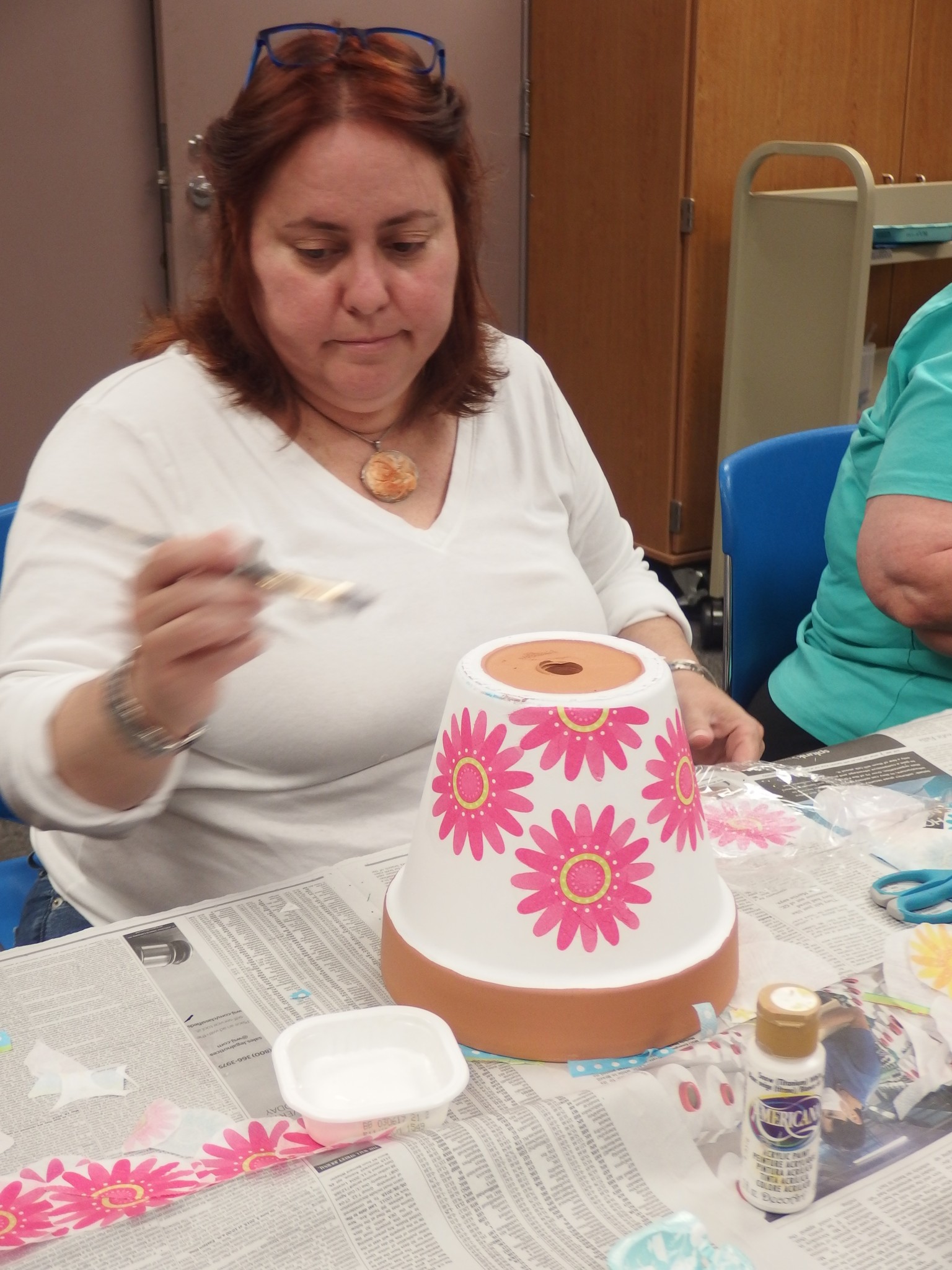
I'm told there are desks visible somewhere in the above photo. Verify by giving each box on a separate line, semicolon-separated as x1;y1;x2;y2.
0;708;951;1270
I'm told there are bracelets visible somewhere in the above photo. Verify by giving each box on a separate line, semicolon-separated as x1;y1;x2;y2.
106;645;209;756
668;659;719;687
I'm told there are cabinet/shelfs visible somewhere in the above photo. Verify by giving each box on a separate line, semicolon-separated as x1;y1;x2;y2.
523;0;952;567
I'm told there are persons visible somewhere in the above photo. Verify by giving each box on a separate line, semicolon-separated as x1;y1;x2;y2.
752;289;952;761
814;991;883;1151
0;18;768;978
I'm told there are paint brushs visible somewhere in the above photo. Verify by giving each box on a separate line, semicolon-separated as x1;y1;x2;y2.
23;499;376;616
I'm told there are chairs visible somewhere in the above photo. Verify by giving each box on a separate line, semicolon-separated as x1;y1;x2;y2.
719;424;858;759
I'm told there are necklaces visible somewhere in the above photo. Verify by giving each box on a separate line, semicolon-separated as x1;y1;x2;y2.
298;389;423;507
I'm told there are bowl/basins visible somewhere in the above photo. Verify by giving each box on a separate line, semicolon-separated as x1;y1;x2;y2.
271;1005;472;1149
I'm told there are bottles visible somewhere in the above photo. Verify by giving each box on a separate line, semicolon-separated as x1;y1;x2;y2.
739;981;827;1215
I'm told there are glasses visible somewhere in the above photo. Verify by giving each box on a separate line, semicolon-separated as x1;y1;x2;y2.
241;24;448;94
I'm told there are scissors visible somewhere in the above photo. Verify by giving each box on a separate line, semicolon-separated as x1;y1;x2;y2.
869;861;951;924
